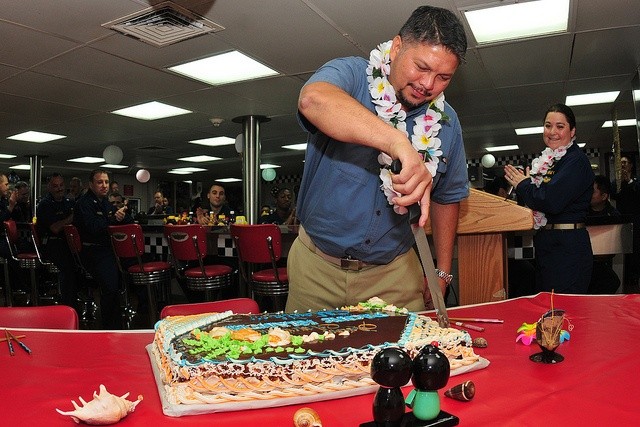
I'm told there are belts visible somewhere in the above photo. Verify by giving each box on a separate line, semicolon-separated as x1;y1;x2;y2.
543;223;586;231
298;224;373;271
49;236;63;239
81;242;101;247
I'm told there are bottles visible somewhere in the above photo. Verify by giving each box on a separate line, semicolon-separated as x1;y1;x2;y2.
269;209;278;225
229;210;236;223
123;199;129;224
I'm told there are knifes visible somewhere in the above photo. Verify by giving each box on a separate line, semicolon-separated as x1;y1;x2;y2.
390;159;452;330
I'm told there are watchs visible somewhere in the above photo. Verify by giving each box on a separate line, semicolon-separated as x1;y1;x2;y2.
433;268;455;285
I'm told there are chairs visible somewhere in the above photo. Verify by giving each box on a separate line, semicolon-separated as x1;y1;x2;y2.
30;224;61;274
162;226;233;302
2;220;39;306
228;223;290;311
158;297;260;322
108;224;170;327
0;304;79;329
64;223;95;281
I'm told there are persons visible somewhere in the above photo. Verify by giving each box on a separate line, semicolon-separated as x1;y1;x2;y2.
79;170;126;307
193;183;237;225
0;176;18;225
591;176;621;294
36;174;75;262
149;190;167;218
108;192;132;216
620;156;633;184
283;4;471;314
269;185;300;232
504;106;597;296
259;206;272;215
13;180;33;241
109;180;120;194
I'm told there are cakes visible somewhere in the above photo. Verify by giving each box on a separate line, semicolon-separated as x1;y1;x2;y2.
146;295;490;417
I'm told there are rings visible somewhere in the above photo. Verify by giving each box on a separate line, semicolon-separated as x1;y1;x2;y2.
509;176;514;180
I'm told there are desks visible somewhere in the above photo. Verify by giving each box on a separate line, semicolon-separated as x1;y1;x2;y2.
0;287;640;427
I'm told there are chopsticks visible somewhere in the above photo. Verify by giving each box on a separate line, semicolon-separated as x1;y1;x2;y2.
431;314;507;331
0;327;31;357
